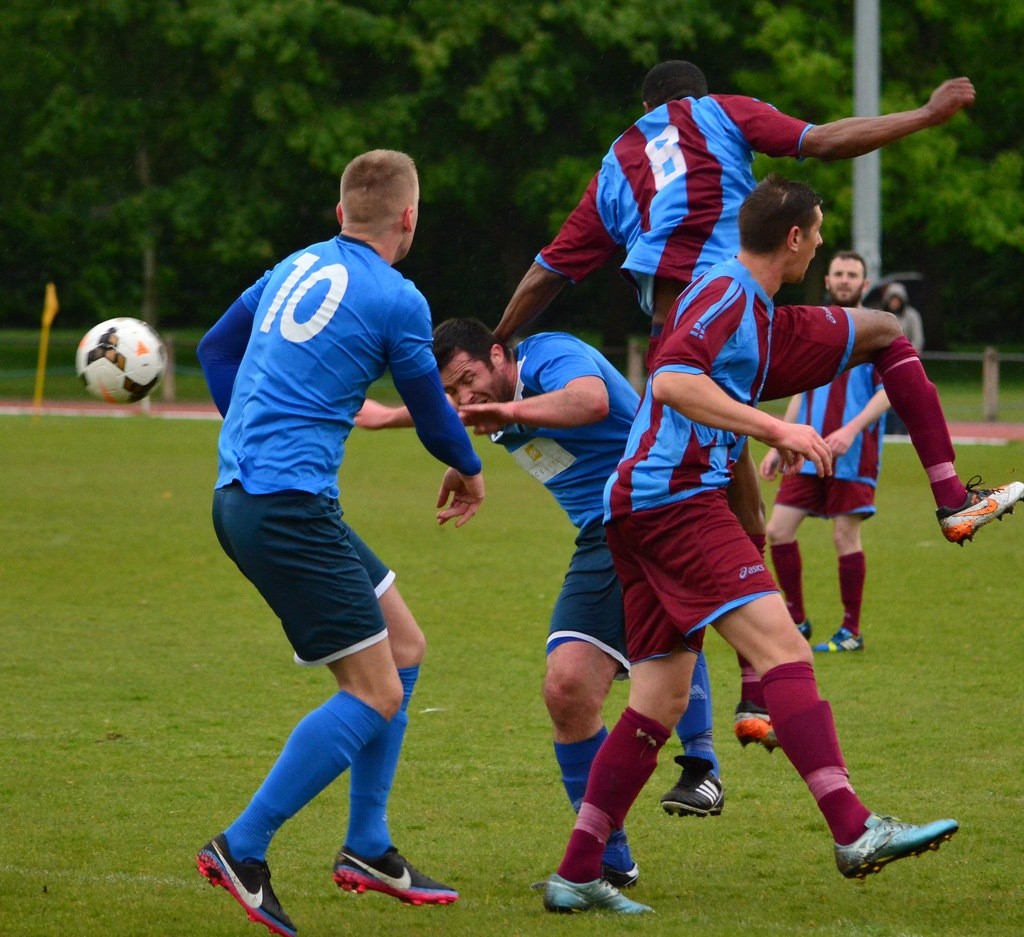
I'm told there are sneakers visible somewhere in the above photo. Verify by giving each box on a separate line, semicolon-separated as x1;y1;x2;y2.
792;621;812;640
333;844;458;906
543;872;657;914
812;626;862;656
937;481;1024;543
734;701;782;753
529;863;640;890
198;832;298;937
662;755;725;817
833;811;959;881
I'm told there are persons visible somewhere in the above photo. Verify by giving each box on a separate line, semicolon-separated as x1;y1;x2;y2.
187;149;484;937
879;282;924;435
489;56;1022;759
548;169;968;917
758;248;897;656
351;314;729;898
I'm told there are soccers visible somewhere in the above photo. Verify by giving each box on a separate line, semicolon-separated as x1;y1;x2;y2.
74;316;167;405
